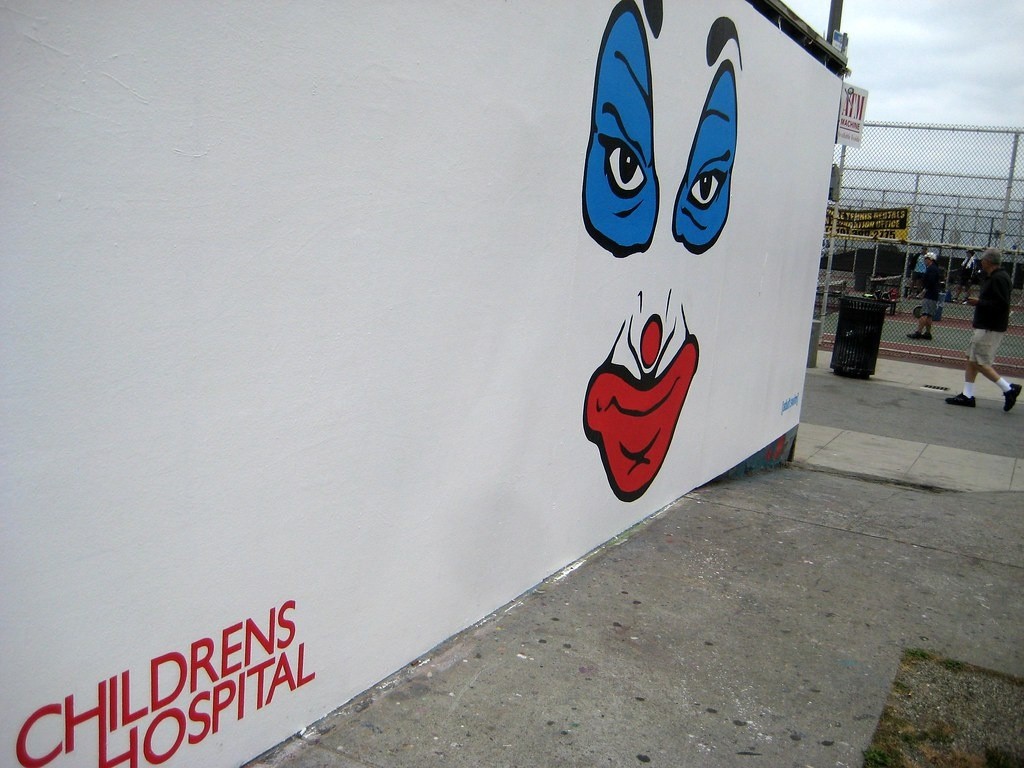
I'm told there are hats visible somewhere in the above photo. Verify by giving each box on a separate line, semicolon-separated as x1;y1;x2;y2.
921;252;937;261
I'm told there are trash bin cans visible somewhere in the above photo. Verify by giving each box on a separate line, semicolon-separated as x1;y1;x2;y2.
829;294;890;381
932;293;946;321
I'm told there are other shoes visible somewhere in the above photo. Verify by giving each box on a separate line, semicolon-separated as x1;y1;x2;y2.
961;300;967;305
952;299;958;303
921;333;932;340
907;332;921;339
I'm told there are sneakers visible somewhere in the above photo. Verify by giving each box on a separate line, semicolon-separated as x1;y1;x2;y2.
1004;383;1022;411
945;393;975;407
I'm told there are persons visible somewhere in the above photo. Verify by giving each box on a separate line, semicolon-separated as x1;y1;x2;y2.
905;246;940;341
944;248;1022;413
